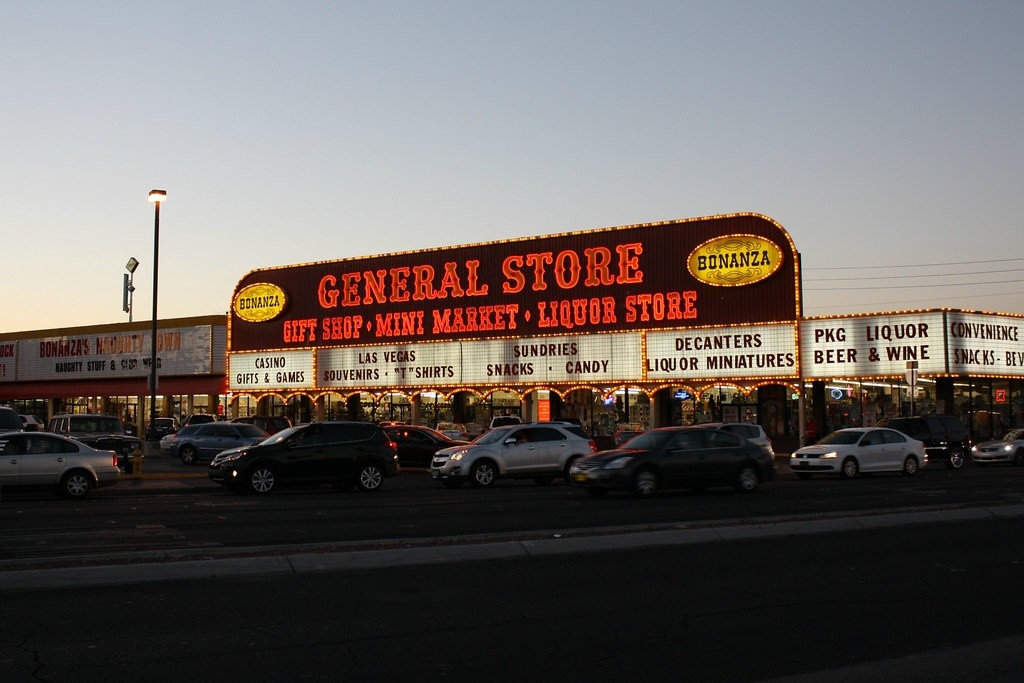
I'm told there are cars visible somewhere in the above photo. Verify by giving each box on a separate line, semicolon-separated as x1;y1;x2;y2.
379;416;596;489
0;406;142;499
146;413;294;466
790;427;928;480
971;429;1024;464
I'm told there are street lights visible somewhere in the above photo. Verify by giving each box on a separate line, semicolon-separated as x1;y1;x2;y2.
144;189;168;457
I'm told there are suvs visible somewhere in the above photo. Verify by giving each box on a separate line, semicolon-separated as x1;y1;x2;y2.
699;423;776;462
872;415;972;469
568;420;776;500
211;421;400;498
